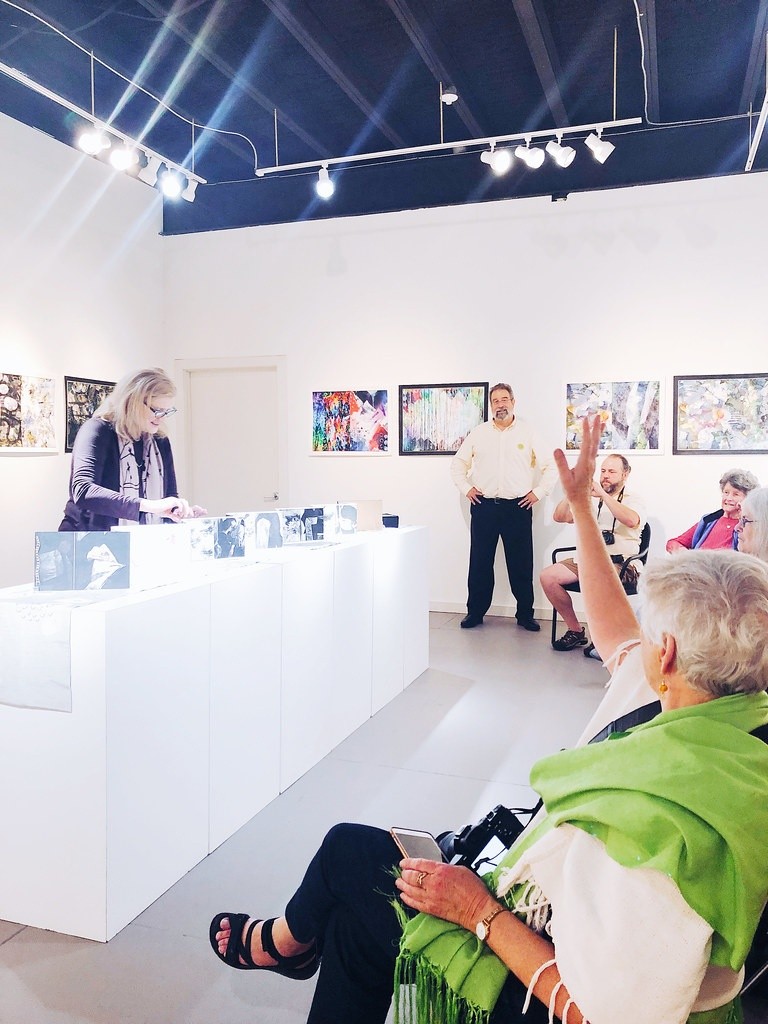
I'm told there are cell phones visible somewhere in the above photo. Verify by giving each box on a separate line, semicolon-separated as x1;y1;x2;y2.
389;827;451;864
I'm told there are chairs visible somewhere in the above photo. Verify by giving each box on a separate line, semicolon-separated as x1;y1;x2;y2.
551;522;651;657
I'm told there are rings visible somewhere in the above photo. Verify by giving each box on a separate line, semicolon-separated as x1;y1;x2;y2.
416;872;427;886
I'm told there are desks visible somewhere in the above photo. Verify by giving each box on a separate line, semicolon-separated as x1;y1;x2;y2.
0;520;432;944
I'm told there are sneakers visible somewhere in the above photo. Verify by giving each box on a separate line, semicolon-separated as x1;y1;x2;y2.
553;626;588;651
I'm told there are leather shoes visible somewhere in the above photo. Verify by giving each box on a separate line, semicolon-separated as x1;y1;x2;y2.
461;614;483;628
517;617;540;631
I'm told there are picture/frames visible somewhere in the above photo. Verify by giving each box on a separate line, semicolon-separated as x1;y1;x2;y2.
0;371;62;453
399;382;489;456
64;376;118;453
673;373;768;454
307;387;394;457
562;376;665;456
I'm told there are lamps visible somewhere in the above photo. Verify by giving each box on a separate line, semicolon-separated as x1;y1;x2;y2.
442;83;459;106
515;137;545;168
78;124;111;154
111;141;139;169
545;133;577;168
584;127;617;164
315;165;334;198
480;142;511;172
162;168;181;198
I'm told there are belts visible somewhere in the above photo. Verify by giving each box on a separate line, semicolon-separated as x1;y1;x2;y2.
478;497;525;504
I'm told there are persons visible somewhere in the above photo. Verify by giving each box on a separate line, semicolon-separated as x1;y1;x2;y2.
59;368;207;531
461;383;560;631
212;416;768;1024
218;520;245;557
538;453;645;652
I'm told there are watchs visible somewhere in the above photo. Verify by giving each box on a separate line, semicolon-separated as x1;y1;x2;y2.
475;907;508;942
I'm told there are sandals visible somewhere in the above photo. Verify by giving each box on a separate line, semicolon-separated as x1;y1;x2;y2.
209;912;320;980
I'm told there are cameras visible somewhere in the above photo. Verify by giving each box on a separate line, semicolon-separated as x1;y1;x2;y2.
602;530;614;544
434;805;526;878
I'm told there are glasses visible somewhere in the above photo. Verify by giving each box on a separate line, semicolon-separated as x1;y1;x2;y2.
491;398;513;404
143;401;177;419
739;518;756;527
